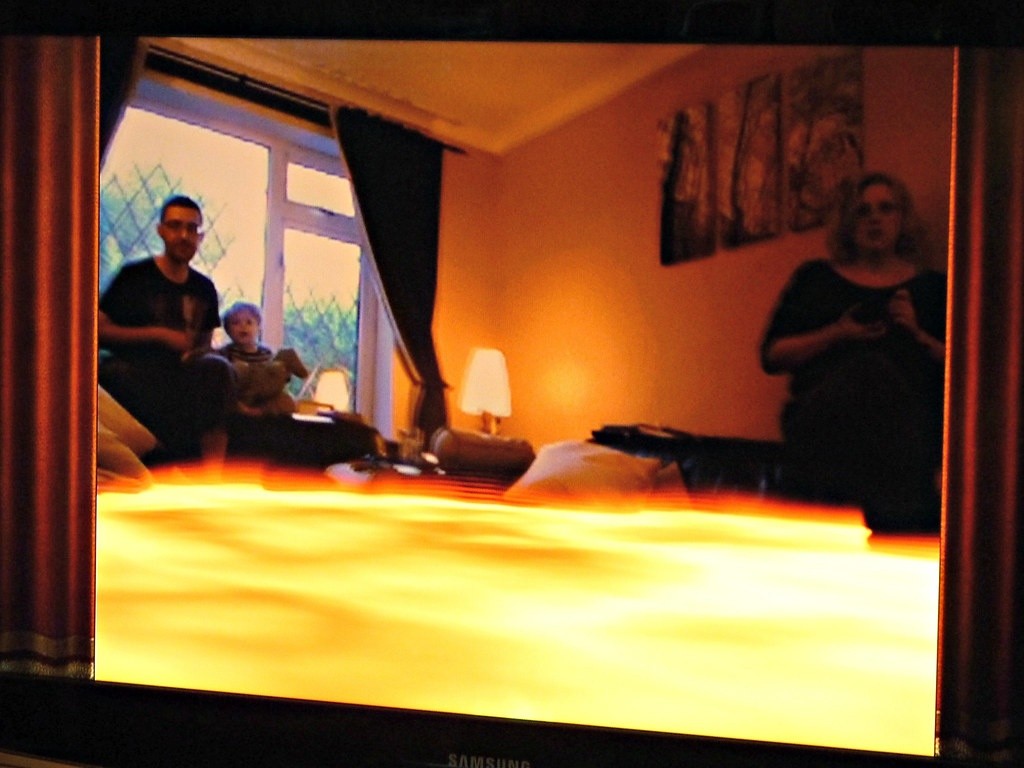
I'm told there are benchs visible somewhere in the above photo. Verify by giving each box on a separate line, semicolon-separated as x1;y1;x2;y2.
595;431;795;497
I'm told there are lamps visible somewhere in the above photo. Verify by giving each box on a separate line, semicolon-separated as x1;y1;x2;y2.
313;370;351;414
462;347;510;436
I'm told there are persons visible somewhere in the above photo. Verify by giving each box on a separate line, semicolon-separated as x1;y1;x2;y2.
218;301;294;445
98;194;231;468
759;173;945;503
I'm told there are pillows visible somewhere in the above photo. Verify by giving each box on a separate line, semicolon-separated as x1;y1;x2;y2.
505;441;662;502
